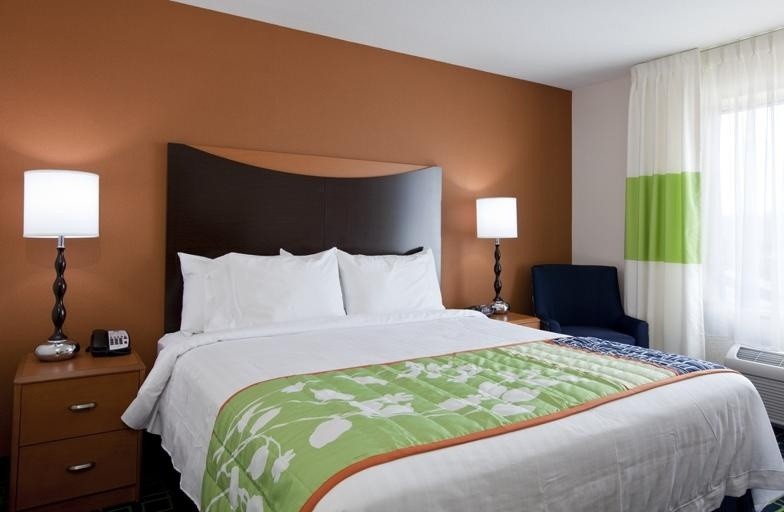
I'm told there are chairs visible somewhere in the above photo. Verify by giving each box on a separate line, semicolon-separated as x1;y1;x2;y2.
530;264;649;349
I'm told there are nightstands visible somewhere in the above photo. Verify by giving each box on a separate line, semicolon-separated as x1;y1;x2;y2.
9;349;148;512
488;311;540;329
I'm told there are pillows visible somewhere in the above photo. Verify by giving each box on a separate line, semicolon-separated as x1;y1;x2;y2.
176;244;447;338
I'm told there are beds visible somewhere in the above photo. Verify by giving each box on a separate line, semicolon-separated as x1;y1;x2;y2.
160;140;763;512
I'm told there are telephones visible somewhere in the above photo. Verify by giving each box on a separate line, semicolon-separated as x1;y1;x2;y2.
90;329;132;357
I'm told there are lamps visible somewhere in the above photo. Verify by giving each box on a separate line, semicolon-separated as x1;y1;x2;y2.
21;168;100;363
475;196;518;314
